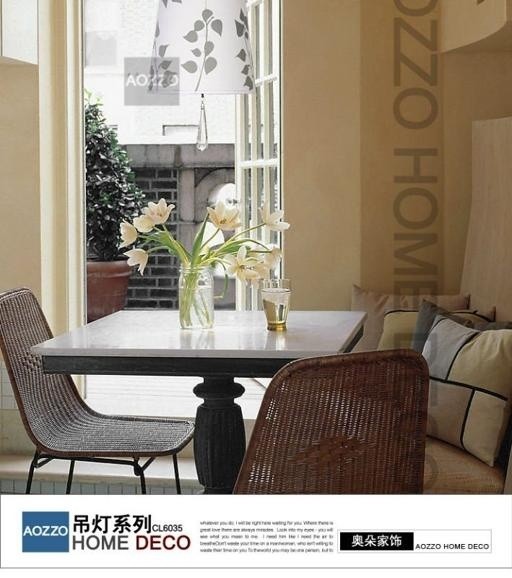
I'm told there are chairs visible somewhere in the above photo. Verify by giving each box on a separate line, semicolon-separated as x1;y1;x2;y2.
0;284;199;494
229;344;430;494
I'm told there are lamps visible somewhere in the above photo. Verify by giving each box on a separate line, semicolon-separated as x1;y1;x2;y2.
141;0;259;152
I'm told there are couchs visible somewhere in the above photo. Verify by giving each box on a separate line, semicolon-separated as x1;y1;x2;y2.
422;432;512;494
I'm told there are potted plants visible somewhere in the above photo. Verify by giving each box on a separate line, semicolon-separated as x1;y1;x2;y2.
81;85;150;321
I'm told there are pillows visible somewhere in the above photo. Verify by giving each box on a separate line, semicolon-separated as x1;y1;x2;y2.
413;313;512;467
345;281;473;354
410;298;512;371
373;304;500;355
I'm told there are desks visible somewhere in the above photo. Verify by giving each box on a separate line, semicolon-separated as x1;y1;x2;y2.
28;306;372;493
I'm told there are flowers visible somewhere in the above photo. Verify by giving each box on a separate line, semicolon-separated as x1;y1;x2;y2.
112;196;292;287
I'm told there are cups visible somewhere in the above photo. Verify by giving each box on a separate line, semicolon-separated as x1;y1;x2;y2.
258;277;292;332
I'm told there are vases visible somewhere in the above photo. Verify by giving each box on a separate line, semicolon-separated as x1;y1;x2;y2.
176;261;216;331
